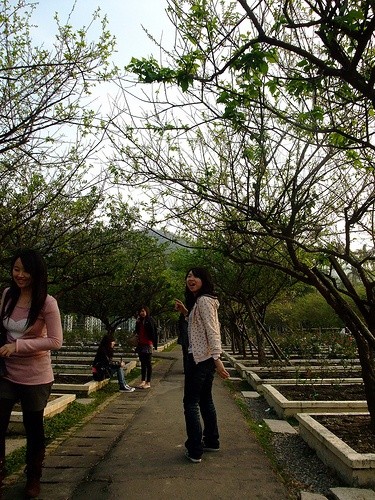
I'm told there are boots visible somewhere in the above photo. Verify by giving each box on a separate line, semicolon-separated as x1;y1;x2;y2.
24;447;45;497
0;456;6;500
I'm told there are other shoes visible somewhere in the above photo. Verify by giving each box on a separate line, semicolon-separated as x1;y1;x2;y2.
201;441;219;452
185;449;201;463
135;384;150;389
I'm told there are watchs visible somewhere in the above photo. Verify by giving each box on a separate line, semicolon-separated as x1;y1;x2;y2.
214;356;220;360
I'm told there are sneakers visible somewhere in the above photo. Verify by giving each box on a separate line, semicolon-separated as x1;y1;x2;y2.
119;386;134;392
125;383;136;390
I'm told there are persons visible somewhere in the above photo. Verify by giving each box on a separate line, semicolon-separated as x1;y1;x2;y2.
175;267;229;463
0;248;63;499
132;306;158;388
178;306;189;373
340;327;352;334
92;334;135;392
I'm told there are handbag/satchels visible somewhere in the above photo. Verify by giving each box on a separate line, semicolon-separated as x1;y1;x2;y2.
93;364;106;381
0;316;7;376
